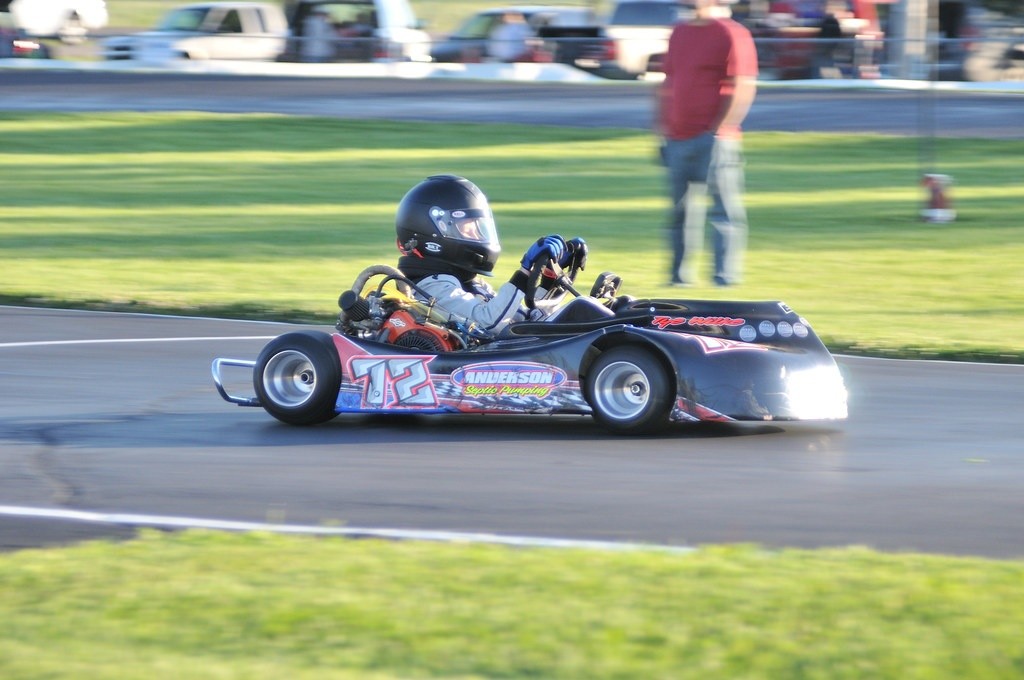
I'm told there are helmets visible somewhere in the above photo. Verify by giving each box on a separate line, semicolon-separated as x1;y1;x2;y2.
397;174;502;276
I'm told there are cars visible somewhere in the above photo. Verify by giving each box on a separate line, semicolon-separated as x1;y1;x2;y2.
274;1;433;63
1;27;51;59
432;4;617;66
103;3;289;61
603;2;704;80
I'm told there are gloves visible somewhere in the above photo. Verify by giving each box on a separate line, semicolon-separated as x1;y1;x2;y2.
520;235;568;272
559;237;588;273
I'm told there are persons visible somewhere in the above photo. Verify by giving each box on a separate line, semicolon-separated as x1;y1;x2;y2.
298;13;348;63
397;173;585;333
657;0;759;292
486;12;538;62
